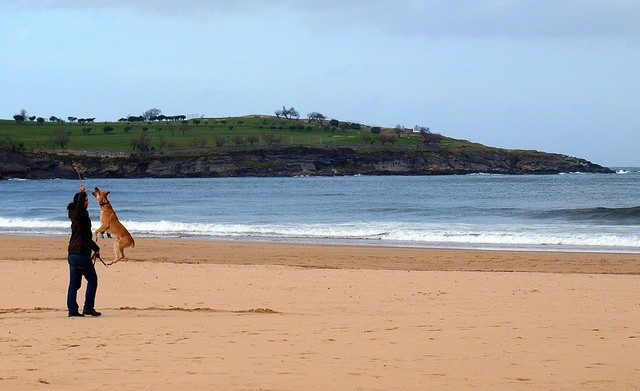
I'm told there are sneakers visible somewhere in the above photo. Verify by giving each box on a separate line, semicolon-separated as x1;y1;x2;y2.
68;311;85;317
83;309;101;316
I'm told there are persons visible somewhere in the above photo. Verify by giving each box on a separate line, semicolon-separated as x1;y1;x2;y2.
67;184;101;317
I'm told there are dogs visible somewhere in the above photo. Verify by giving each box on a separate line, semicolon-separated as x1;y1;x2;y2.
91;186;135;266
